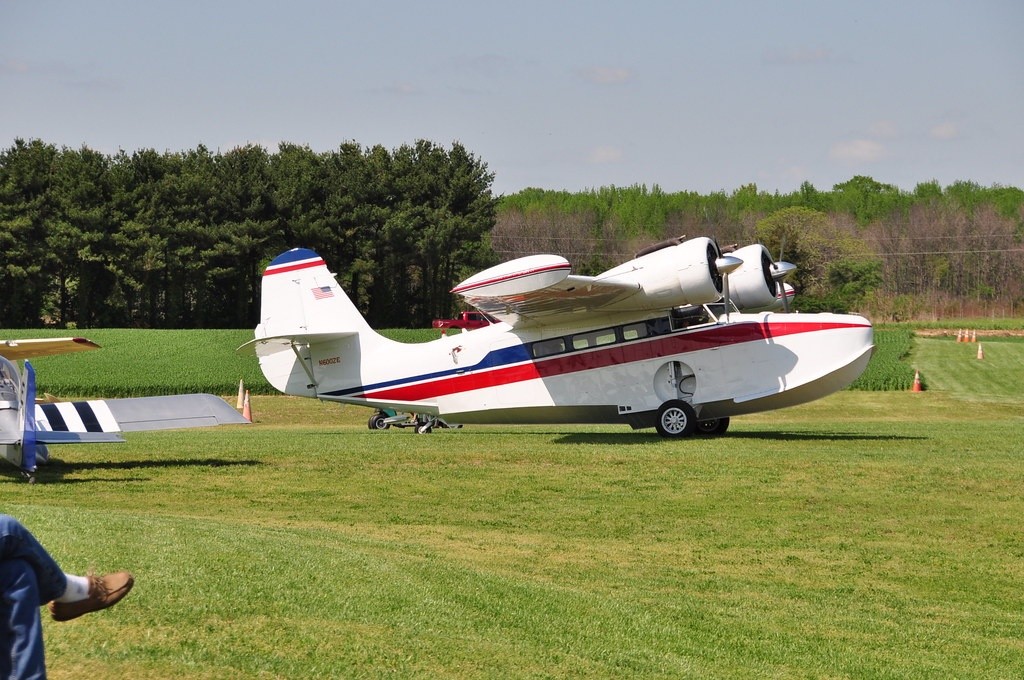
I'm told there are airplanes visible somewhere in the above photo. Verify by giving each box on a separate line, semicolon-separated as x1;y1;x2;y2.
236;234;875;439
0;337;251;484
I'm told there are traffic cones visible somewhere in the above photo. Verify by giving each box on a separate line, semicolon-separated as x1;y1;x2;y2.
971;329;977;342
910;369;924;392
977;343;983;360
957;328;962;342
236;379;246;409
243;390;252;422
964;329;969;342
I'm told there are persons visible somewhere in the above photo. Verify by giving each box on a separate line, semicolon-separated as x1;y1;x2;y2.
0;514;134;680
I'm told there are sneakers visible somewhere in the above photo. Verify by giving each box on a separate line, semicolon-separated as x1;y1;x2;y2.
48;572;134;622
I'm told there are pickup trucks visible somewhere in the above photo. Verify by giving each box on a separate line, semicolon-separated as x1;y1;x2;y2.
432;312;502;334
